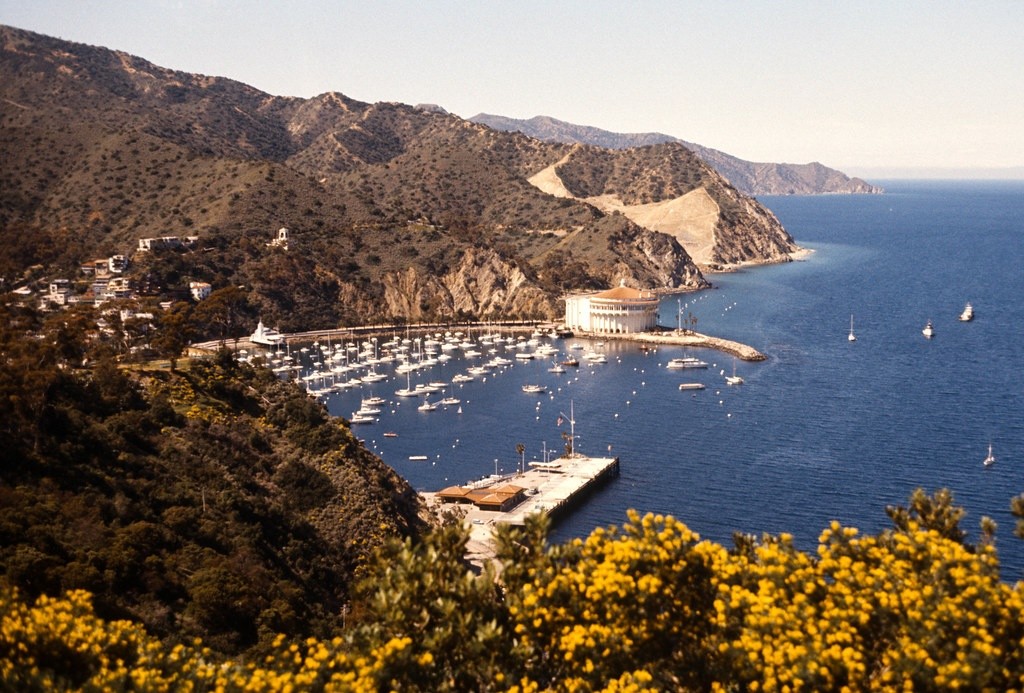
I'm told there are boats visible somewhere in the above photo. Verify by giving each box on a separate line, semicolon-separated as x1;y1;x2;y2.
959;302;973;321
237;319;744;430
983;445;995;465
922;324;935;338
848;314;857;341
